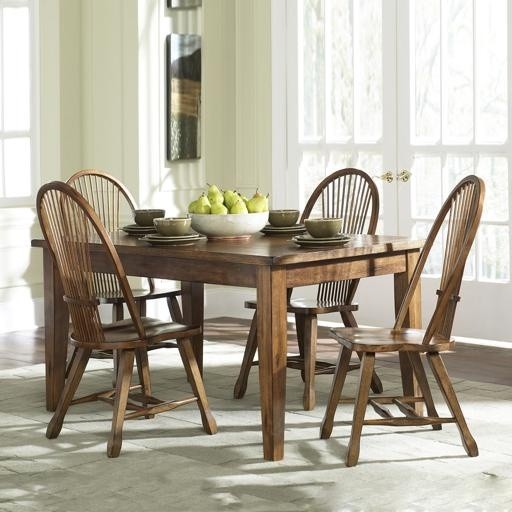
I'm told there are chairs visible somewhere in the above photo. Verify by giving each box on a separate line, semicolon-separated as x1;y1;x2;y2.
318;168;486;467
232;164;384;409
58;168;183;387
35;180;218;459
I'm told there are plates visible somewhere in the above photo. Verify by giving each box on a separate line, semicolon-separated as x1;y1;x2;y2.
127;224;156;229
123;227;157;235
260;228;307;236
264;223;305;229
139;234;207;247
294;234;347;240
292;236;354;249
145;231;200;240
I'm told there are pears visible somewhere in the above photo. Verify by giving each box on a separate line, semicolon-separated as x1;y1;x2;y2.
189;184;270;214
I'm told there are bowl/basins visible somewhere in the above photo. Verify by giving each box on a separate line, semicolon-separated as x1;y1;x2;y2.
269;210;299;226
303;217;344;238
153;217;192;236
187;211;270;239
134;209;166;226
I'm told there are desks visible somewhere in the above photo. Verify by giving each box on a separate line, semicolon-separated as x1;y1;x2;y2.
30;234;429;461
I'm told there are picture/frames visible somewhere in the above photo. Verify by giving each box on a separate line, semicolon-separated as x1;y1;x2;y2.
167;31;203;163
168;0;203;9
